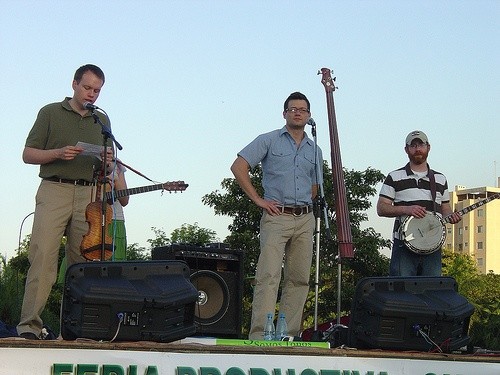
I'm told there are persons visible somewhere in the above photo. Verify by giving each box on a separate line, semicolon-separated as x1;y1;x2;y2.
17;64;128;339
231;92;324;342
377;132;461;276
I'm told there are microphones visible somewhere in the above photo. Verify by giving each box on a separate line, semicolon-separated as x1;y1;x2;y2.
83;102;99;109
307;118;316;126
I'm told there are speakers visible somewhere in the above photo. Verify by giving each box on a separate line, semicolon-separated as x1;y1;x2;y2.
61;260;199;343
346;276;475;354
152;243;245;339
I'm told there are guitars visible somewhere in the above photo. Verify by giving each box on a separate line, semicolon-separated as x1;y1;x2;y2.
104;180;189;202
397;192;500;256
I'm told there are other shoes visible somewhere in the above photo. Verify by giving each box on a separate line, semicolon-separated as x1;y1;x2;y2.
20;332;36;340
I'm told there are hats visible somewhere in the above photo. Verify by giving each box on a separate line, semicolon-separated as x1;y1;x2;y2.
406;131;428;145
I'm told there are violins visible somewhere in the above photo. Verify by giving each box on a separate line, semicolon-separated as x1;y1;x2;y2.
80;161;117;261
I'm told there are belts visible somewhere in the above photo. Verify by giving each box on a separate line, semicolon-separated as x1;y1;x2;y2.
44;177;91;186
271;206;312;217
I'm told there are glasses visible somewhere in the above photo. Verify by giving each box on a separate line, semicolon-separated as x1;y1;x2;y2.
286;108;309;113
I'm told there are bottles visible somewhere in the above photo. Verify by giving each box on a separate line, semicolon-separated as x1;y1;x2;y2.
264;313;275;341
276;313;288;341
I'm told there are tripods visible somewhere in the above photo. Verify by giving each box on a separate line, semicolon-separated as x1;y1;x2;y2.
323;244;351;348
